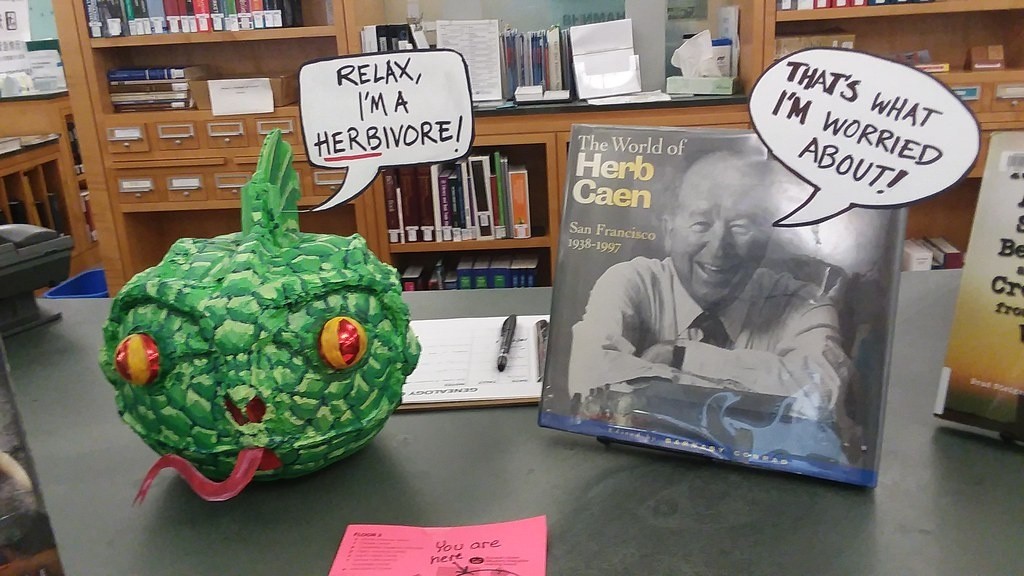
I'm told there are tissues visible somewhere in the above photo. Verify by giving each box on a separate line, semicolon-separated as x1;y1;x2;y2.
665;29;738;94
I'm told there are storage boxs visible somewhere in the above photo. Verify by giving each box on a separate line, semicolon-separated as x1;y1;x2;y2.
41;268;111;299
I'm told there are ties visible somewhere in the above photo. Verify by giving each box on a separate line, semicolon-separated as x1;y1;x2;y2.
687;312;729;348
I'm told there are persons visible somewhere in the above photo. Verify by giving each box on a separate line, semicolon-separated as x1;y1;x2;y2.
568;149;852;466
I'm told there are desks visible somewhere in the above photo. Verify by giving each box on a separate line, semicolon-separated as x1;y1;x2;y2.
20;266;1024;576
0;88;97;259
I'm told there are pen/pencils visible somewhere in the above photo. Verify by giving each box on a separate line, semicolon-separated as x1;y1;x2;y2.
495;313;519;372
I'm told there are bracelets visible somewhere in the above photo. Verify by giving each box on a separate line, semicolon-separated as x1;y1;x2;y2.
671;345;685;370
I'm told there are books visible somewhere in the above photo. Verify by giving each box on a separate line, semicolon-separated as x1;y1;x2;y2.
0;137;22;155
902;236;962;270
81;190;99;242
360;23;430;53
0;194;65;236
776;0;946;11
500;29;577;99
402;254;539;291
394;315;553;410
380;152;529;243
83;0;304;39
108;65;209;113
66;122;85;175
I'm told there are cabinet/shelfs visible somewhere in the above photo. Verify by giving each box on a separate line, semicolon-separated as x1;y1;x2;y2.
52;0;1023;296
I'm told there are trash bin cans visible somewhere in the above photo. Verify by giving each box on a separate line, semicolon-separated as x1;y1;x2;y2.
44;268;110;298
